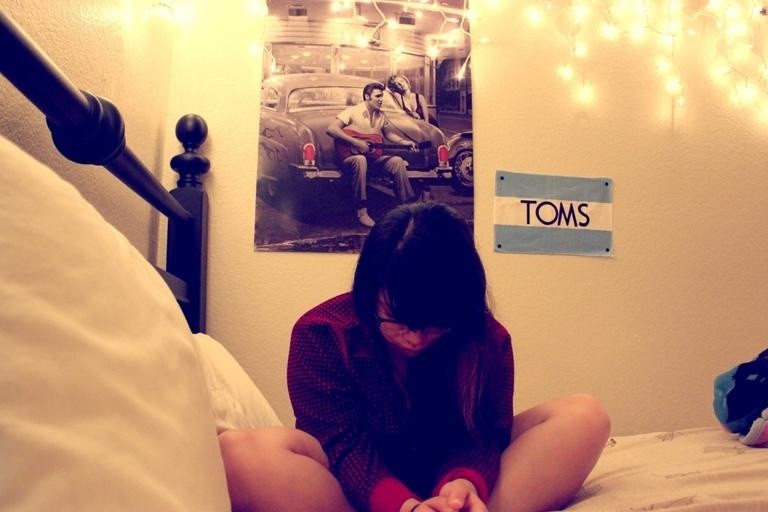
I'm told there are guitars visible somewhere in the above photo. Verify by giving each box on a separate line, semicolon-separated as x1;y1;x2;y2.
334;129;433;160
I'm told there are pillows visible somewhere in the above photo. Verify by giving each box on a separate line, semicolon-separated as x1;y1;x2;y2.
0;137;239;512
191;329;283;435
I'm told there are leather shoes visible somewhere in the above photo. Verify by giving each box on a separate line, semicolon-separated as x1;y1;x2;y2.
358;211;376;228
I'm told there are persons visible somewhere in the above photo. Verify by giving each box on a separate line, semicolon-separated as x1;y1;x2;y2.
327;82;421;227
217;201;611;511
388;75;440;127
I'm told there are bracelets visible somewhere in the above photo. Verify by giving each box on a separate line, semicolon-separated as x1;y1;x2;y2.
410;504;420;511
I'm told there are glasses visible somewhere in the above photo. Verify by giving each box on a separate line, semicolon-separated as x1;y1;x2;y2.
373;306;454;337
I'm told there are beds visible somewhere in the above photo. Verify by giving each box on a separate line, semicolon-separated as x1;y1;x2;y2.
0;6;768;512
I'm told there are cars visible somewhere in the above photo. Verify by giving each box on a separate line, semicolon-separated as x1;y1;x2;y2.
447;130;473;197
257;73;452;202
